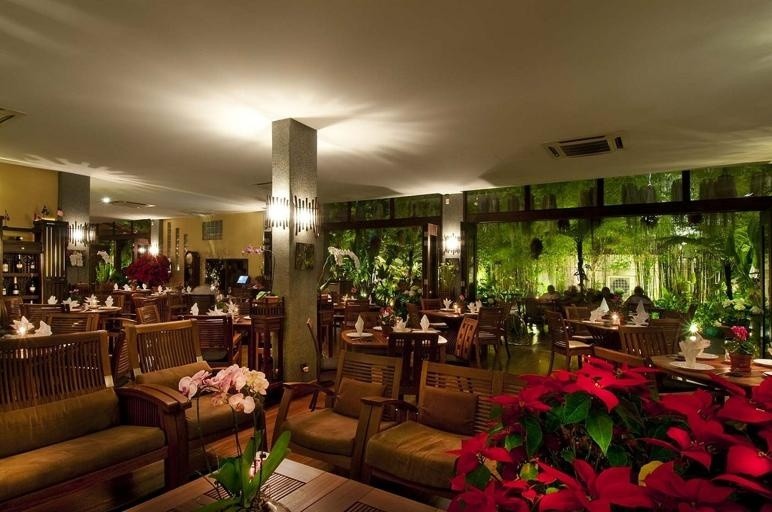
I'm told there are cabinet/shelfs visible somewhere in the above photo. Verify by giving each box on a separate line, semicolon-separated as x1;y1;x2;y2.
1;225;42;304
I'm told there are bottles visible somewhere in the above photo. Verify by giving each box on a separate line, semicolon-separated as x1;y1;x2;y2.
1;279;6;296
11;276;20;296
3;257;8;273
26;274;36;295
14;253;23;273
29;254;36;273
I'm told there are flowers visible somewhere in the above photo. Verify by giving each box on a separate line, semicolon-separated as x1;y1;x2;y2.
439;354;771;511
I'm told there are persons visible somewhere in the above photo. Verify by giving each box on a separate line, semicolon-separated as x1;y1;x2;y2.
246;275;267;299
395;280;410;297
595;287;614;303
623;285;654;309
539;284;561;300
191;276;218;295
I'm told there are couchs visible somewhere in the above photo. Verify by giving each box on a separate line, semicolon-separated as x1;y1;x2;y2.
0;330;177;510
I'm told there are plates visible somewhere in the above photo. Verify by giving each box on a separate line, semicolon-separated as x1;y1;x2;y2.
673;361;715;371
677;351;719;360
441;309;457;312
348;331;374;339
753;358;772;366
414;331;441;334
